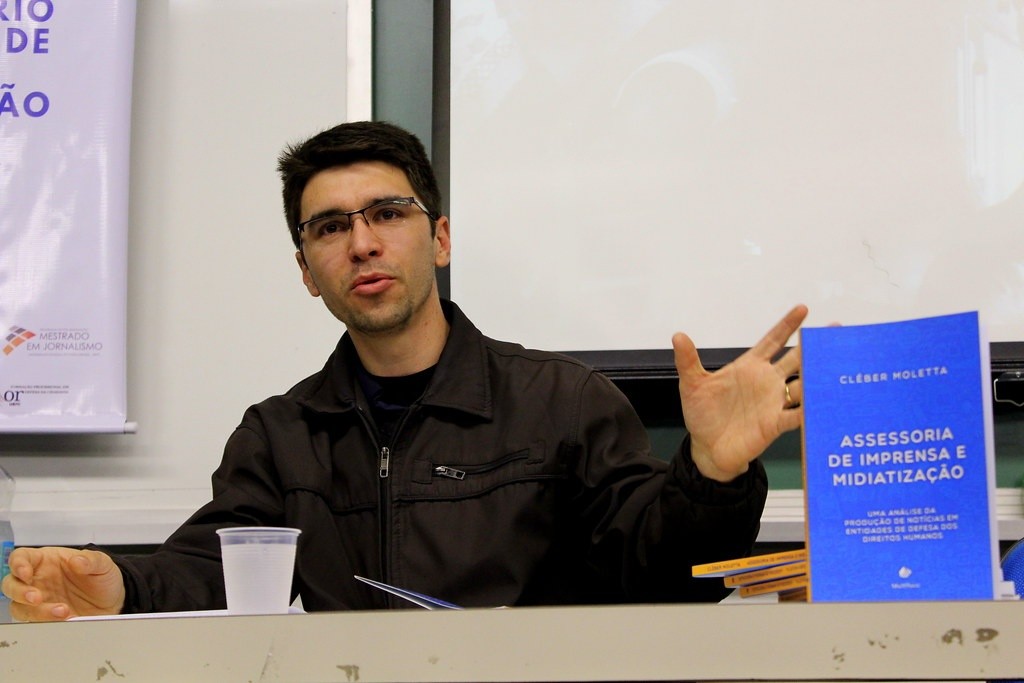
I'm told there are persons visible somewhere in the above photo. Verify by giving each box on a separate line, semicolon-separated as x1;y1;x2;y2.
0;121;843;622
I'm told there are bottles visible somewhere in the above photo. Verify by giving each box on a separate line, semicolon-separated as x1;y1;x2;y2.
0;470;17;625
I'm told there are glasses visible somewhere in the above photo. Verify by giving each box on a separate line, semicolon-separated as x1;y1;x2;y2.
297;197;432;250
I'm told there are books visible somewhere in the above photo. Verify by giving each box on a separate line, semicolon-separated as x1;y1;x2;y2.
691;309;1020;604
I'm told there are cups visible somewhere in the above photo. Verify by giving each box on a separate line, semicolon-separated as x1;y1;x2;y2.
215;526;302;613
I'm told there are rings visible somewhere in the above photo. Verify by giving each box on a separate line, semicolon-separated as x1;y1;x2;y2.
786;384;793;405
